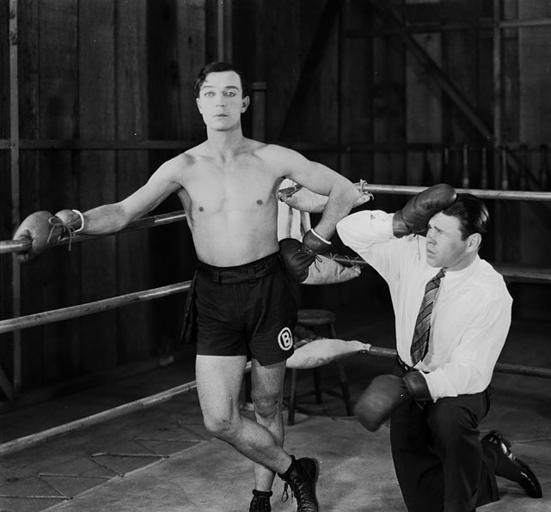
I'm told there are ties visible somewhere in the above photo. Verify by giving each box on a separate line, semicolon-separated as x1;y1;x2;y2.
410;268;446;366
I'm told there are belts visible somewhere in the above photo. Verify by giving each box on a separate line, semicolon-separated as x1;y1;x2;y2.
396;354;418;372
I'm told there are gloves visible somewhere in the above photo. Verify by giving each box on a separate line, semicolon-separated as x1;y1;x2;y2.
352;371;432;433
11;208;85;265
392;182;457;237
278;229;332;286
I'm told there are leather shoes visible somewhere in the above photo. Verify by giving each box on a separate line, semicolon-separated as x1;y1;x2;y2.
479;429;543;498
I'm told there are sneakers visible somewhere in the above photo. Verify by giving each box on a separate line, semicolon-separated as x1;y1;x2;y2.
249;489;273;512
278;454;320;511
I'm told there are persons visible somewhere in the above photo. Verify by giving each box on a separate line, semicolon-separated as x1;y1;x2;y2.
333;181;543;512
10;59;364;511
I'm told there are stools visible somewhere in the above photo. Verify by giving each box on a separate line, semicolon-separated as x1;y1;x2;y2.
289;309;355;426
